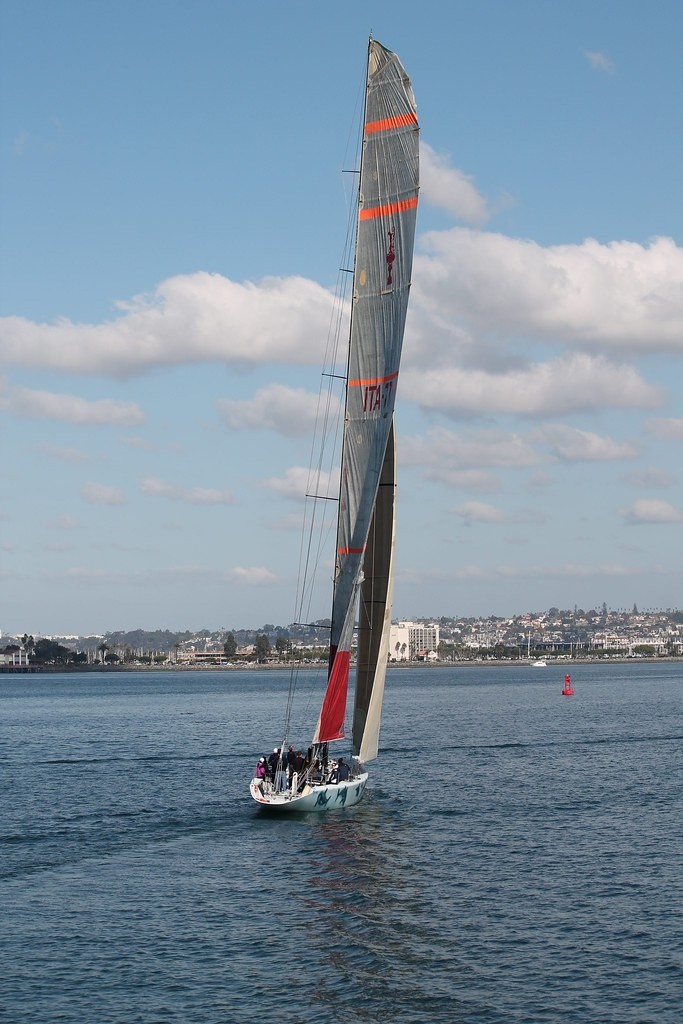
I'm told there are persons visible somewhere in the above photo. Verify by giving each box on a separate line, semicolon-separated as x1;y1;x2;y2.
326;757;351;784
256;744;306;793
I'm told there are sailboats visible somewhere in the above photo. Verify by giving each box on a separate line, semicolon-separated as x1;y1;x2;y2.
248;33;422;812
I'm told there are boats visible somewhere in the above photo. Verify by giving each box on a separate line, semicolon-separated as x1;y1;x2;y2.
530;661;547;667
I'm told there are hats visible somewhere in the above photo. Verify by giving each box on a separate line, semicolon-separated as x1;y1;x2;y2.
259;758;265;763
288;745;294;750
296;751;303;754
273;748;278;754
